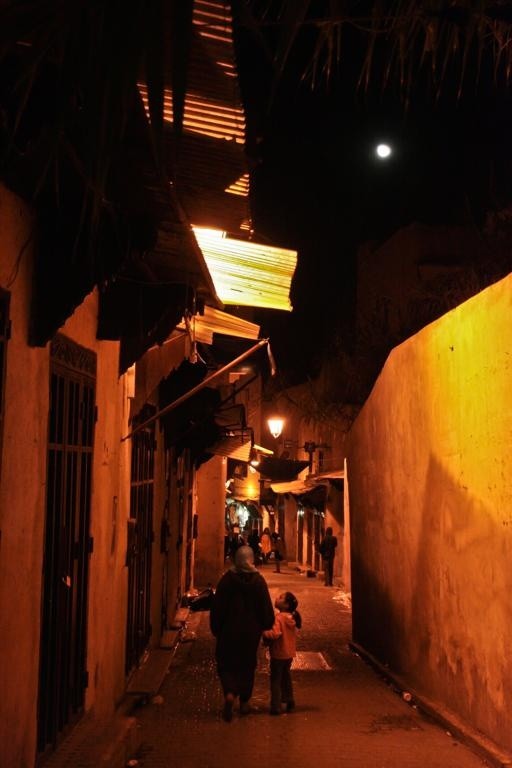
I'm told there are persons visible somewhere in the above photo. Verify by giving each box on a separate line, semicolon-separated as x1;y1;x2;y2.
209;545;276;720
263;591;302;715
319;524;340;588
230;521;285;575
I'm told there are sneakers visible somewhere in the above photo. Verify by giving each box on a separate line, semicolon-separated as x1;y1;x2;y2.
240;703;259;715
286;701;295;712
270;706;281;714
224;693;234;722
